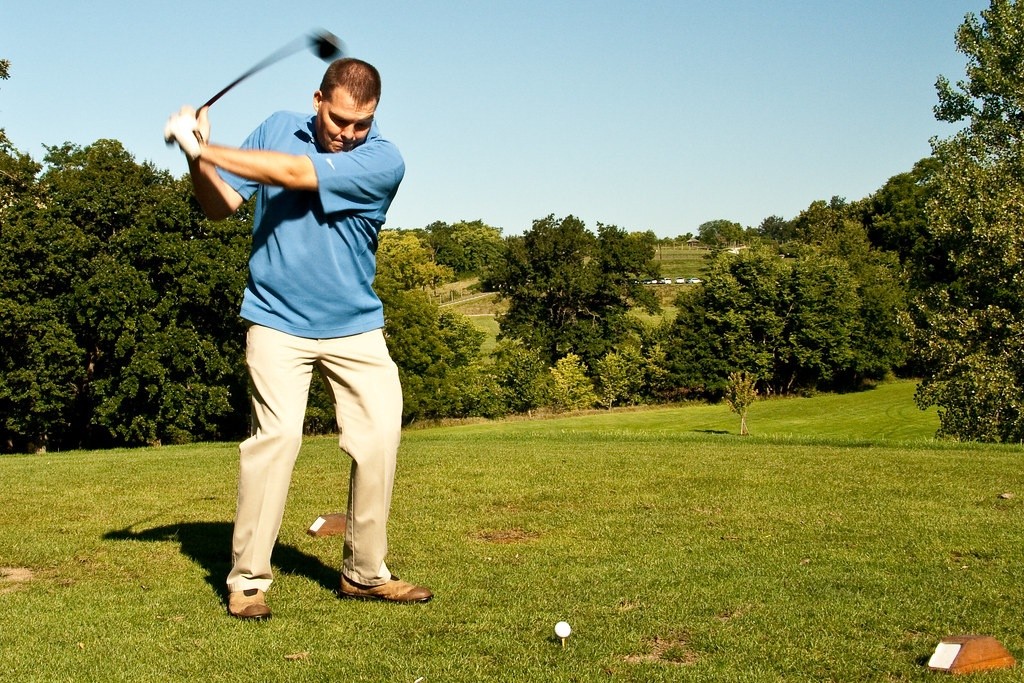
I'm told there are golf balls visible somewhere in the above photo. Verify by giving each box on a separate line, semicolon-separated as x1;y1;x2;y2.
554;621;570;638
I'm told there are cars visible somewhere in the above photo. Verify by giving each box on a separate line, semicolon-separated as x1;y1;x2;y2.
647;277;672;285
674;277;685;284
686;278;703;284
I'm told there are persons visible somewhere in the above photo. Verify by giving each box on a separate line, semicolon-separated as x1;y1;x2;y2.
165;58;434;621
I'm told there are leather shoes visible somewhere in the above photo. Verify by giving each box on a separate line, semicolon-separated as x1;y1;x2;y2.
227;589;272;623
341;568;434;604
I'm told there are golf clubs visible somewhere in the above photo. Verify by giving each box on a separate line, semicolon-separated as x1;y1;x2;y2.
162;27;348;148
